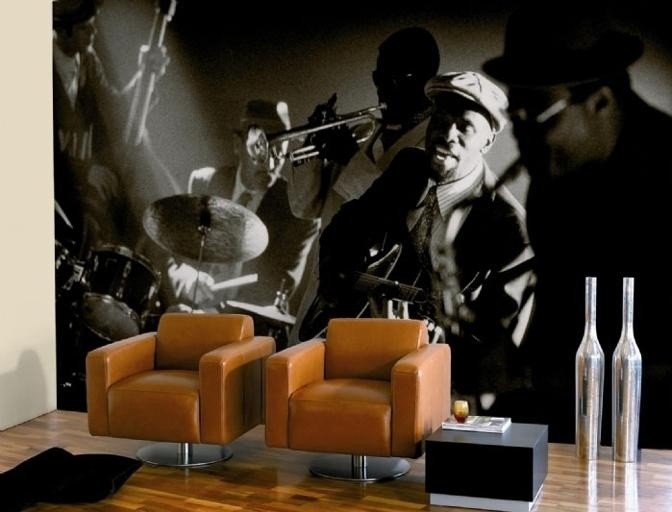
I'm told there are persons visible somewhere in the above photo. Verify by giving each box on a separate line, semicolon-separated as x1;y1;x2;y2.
161;98;321;309
287;25;439;351
479;4;671;450
301;71;536;416
54;2;182;272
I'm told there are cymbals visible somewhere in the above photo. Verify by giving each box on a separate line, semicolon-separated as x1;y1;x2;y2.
228;300;296;324
141;193;268;265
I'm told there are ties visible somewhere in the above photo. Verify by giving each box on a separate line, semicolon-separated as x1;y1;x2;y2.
203;193;254;300
410;183;441;255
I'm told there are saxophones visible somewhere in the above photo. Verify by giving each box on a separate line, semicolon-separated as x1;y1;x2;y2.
431;161;521;413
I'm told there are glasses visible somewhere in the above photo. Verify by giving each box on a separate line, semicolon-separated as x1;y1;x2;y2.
501;88;593;132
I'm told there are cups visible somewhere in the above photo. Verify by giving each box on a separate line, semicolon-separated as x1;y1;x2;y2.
453;400;469;423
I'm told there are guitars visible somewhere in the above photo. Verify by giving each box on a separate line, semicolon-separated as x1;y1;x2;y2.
299;241;467;341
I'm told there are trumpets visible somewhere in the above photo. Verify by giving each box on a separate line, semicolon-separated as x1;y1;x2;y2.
247;102;385;164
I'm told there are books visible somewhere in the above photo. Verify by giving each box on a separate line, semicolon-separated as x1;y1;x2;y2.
441;414;512;434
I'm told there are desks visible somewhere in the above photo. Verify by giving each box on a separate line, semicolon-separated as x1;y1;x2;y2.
423;416;548;510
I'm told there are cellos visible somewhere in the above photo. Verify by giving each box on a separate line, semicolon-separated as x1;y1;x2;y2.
79;4;181;254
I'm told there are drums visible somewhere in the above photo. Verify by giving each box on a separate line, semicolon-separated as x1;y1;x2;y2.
73;245;163;345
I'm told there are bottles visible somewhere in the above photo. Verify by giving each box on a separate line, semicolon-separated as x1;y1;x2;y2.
577;459;599;511
610;458;639;512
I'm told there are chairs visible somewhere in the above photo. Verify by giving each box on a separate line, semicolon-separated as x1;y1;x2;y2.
83;311;282;472
254;318;454;483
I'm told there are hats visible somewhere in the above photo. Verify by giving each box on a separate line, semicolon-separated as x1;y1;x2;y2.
53;1;104;31
478;1;648;91
228;99;297;140
423;69;510;135
